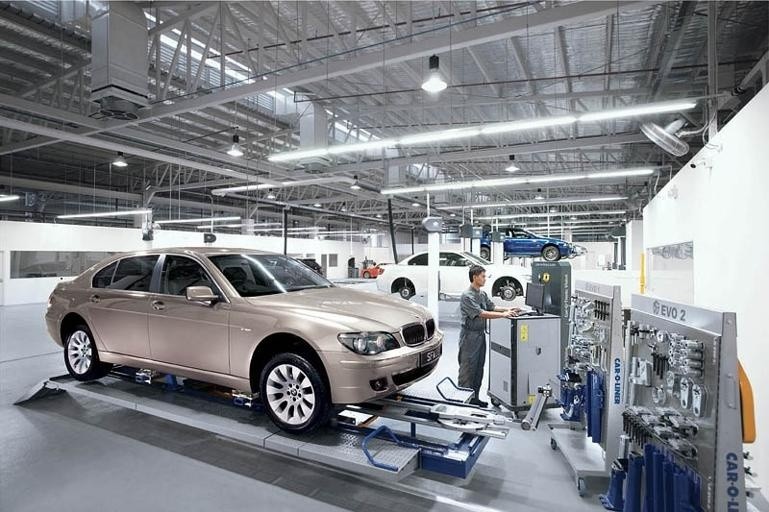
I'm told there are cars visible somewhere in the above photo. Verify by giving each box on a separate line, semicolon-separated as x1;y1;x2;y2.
480;227;588;262
376;249;531;301
361;263;386;279
265;258;323;286
45;247;444;435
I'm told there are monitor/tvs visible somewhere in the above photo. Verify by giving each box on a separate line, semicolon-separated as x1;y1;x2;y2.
525;283;546;316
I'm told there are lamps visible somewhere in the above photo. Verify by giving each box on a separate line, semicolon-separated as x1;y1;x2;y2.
265;175;635;236
267;99;701;164
421;55;447;97
112;151;128;167
505;155;520;173
227;135;243;158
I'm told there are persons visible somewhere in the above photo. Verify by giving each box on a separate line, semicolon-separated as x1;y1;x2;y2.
456;264;522;408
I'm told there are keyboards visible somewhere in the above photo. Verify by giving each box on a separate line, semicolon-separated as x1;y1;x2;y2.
517;310;527;316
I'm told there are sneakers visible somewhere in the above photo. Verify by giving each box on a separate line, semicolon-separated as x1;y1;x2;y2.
469;400;487;407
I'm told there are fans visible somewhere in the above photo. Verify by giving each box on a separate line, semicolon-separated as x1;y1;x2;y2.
641;116;698;156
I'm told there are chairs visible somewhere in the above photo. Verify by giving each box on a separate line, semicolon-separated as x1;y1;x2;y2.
168;263;212;295
225;267;272;294
